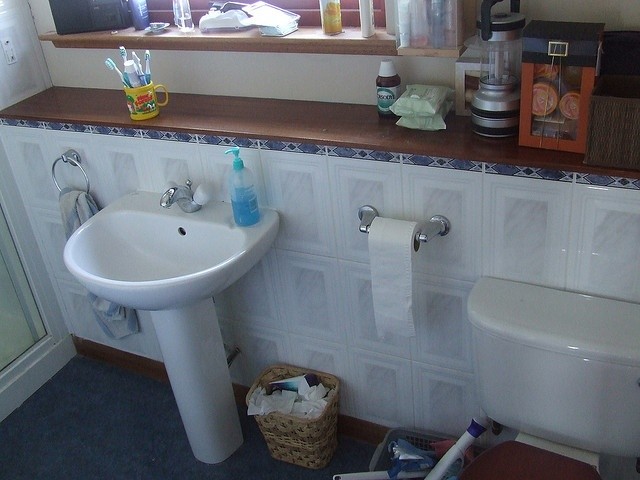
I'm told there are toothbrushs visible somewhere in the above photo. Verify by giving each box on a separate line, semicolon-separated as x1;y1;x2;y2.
130;51;147;84
118;45;130;66
104;57;125;87
142;49;156;84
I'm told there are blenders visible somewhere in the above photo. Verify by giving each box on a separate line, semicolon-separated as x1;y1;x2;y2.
472;0;525;138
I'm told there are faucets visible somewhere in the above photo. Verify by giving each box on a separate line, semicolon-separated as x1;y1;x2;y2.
151;177;213;214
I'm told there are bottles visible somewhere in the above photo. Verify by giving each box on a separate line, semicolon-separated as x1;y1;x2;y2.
375;59;401;117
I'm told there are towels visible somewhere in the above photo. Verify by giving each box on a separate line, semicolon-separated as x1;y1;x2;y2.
59;188;140;340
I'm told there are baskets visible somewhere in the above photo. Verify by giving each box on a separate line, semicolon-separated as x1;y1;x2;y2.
369;426;486;480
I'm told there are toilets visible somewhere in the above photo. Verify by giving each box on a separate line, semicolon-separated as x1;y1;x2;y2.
451;269;640;479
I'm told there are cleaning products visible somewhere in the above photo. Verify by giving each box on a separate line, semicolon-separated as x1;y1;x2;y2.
319;0;342;36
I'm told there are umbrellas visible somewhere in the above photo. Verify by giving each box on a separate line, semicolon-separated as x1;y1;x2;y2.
430;0;444;48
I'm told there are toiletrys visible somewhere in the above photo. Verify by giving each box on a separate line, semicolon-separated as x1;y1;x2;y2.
411;2;429;48
446;0;457;48
397;0;410;49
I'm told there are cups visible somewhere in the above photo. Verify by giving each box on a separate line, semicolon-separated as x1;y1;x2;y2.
124;83;168;121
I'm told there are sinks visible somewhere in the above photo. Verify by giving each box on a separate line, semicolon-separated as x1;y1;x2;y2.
57;193;280;307
